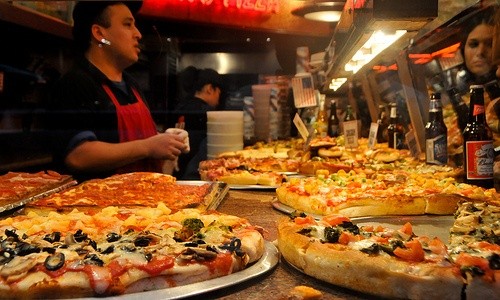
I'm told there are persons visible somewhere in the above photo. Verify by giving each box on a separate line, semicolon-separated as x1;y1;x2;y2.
57;0;186;181
458;14;500;130
168;68;224;180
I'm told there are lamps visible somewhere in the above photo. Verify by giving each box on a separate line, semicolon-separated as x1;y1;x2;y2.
344;30;408;73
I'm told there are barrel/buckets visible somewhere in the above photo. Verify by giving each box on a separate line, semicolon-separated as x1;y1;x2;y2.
252;85;291;140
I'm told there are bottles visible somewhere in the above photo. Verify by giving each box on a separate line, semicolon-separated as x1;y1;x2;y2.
345;105;355;121
448;87;472;132
463;85;495;189
387;103;403;149
425;91;448;164
378;104;388;142
328;99;339;138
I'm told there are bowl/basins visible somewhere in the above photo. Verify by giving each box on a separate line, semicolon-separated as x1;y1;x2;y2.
206;110;244;158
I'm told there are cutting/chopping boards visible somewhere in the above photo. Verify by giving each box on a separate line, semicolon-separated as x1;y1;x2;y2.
271;197;323;219
90;241;279;299
350;216;456;241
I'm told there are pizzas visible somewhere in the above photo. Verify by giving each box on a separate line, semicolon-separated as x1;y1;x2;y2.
0;201;264;300
278;197;500;299
199;134;500;218
25;172;219;210
0;170;74;206
